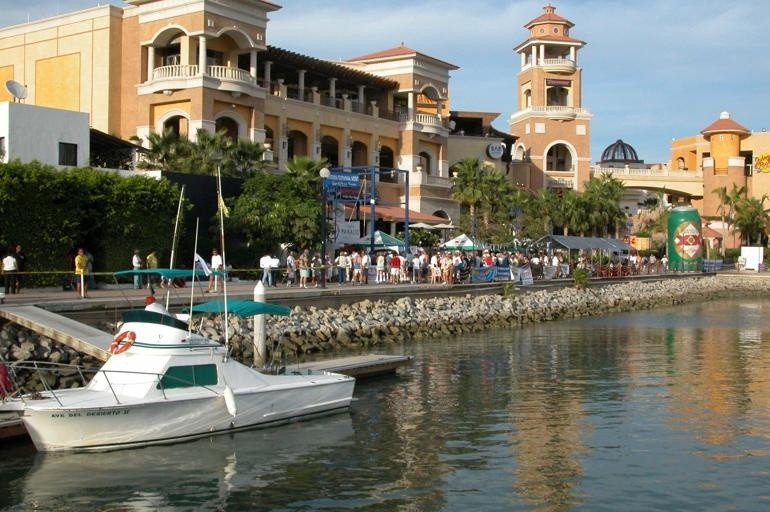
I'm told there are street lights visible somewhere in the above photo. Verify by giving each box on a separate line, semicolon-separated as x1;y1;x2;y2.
318;165;332;288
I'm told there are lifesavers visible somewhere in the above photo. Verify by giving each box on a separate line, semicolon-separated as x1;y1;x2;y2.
110;330;135;353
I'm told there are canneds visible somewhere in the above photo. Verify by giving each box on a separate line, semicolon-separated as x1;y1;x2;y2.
668;207;702;272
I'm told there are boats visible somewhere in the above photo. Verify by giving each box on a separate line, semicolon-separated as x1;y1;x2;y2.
13;417;363;511
0;162;359;458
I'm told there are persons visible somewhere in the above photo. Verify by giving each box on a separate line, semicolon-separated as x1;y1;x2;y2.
74;248;89;298
649;253;656;273
661;254;668;273
0;251;19;295
12;243;28;290
83;248;97;292
63;248;77;291
132;248;144;291
146;249;159;290
203;247;225;294
259;249;649;288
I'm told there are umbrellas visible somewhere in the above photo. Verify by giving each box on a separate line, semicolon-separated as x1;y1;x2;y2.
404;223;436;231
434;223;460;240
